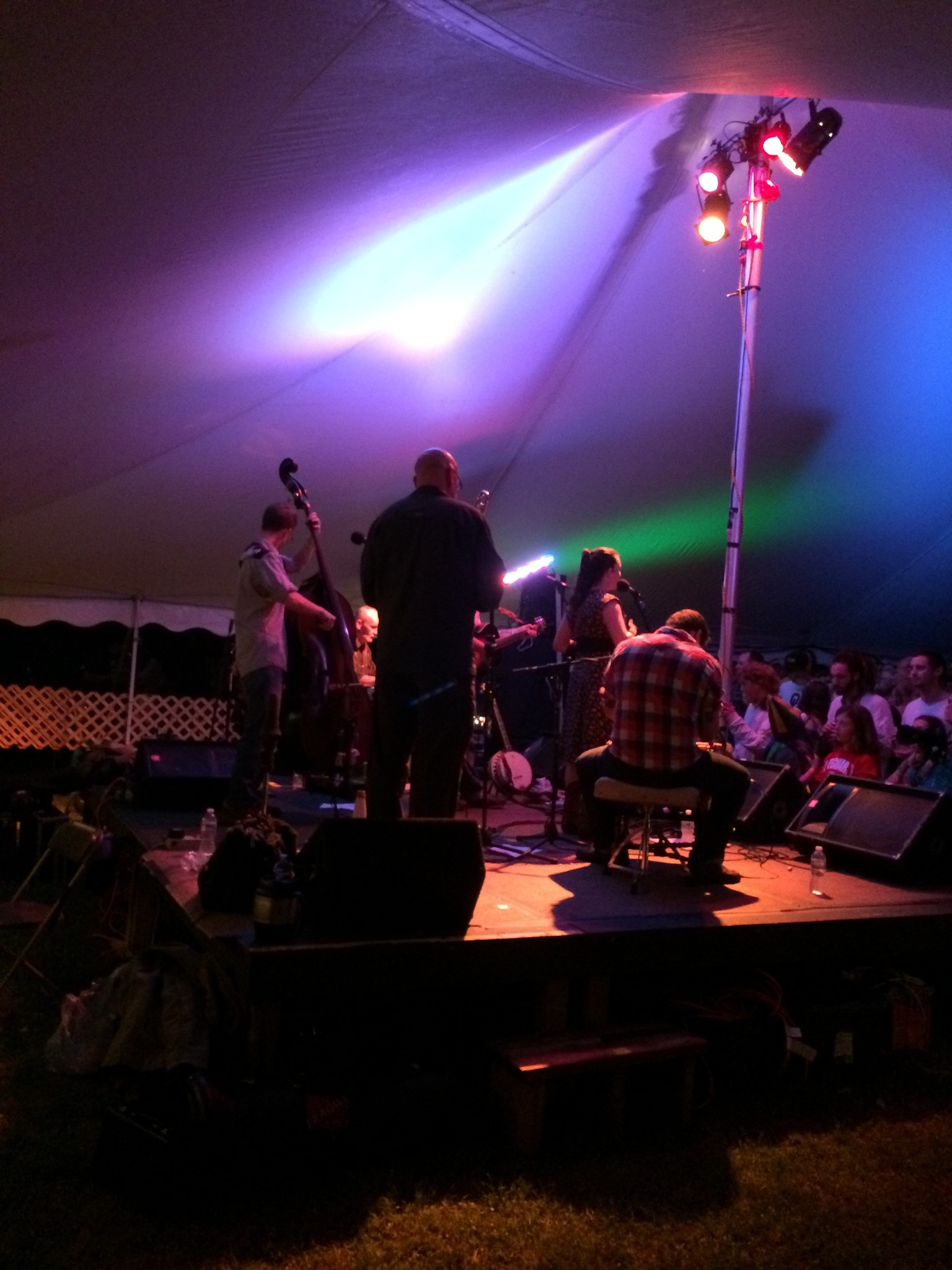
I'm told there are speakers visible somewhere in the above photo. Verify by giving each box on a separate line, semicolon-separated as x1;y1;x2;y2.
133;737;239;816
265;818;485;943
724;756;815;844
783;773;952;890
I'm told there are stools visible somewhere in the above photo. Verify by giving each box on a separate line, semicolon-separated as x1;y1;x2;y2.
591;776;704;894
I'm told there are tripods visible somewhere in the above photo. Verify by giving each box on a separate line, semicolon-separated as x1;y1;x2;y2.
510;661;593;860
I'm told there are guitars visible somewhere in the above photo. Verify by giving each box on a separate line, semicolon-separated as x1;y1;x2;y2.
480;682;532;794
496;616;547;649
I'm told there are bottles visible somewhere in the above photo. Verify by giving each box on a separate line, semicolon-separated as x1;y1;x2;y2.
199;808;217;856
352;791;367;818
809;846;825;896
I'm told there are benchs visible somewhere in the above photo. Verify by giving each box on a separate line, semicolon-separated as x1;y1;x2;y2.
494;1031;704;1159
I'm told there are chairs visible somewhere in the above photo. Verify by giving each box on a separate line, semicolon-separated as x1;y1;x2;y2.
0;776;127;997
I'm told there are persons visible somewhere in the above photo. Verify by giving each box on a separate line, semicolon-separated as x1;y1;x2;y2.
552;547;637;842
353;605;377;686
234;502;338;819
361;449;506;817
576;609;751;883
471;610;544;712
720;648;952;795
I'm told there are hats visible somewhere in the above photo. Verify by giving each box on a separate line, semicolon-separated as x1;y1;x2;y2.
784;650;810;672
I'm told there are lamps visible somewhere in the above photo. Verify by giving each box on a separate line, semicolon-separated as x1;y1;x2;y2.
696;95;842;244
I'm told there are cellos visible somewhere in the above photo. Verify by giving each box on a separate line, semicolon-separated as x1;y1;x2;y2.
279;457;372;814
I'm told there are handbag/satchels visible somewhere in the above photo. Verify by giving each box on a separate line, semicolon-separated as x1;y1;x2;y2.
199;813;296;914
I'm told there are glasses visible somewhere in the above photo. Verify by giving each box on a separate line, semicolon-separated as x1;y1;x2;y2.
453;469;463;491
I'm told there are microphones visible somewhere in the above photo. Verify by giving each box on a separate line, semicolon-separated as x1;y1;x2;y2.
616;579;641;599
351;532;367;545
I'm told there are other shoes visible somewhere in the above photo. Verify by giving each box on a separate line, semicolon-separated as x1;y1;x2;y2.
561;810;593;835
470;790;499;807
682;863;741;887
574;845;631;865
